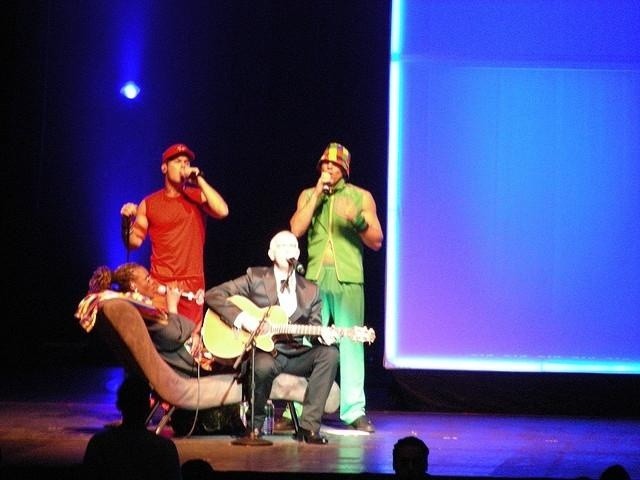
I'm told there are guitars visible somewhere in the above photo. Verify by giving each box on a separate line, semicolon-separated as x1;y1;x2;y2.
202;295;376;359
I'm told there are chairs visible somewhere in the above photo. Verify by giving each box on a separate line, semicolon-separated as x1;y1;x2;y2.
92;295;341;439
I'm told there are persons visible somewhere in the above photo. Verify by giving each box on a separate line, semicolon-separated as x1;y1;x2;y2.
90;262;297;434
195;230;340;446
290;141;384;433
117;141;231;430
0;423;435;479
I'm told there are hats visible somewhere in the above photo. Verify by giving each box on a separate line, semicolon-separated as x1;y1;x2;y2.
162;144;195;163
316;143;351;180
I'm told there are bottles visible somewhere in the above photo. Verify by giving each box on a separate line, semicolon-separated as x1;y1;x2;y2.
262;399;274;435
240;401;248;427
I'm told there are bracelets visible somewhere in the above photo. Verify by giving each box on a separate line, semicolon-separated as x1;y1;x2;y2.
124;226;135;236
350;216;369;232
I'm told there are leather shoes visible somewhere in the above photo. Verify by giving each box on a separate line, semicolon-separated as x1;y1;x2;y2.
246;426;262;441
297;426;325;443
352;414;375;432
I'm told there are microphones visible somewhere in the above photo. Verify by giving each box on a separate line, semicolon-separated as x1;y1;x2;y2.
189;173;196;179
323;184;330;193
287;255;305;275
157;284;196;301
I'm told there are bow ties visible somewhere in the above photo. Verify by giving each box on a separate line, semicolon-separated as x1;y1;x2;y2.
281;280;290;293
